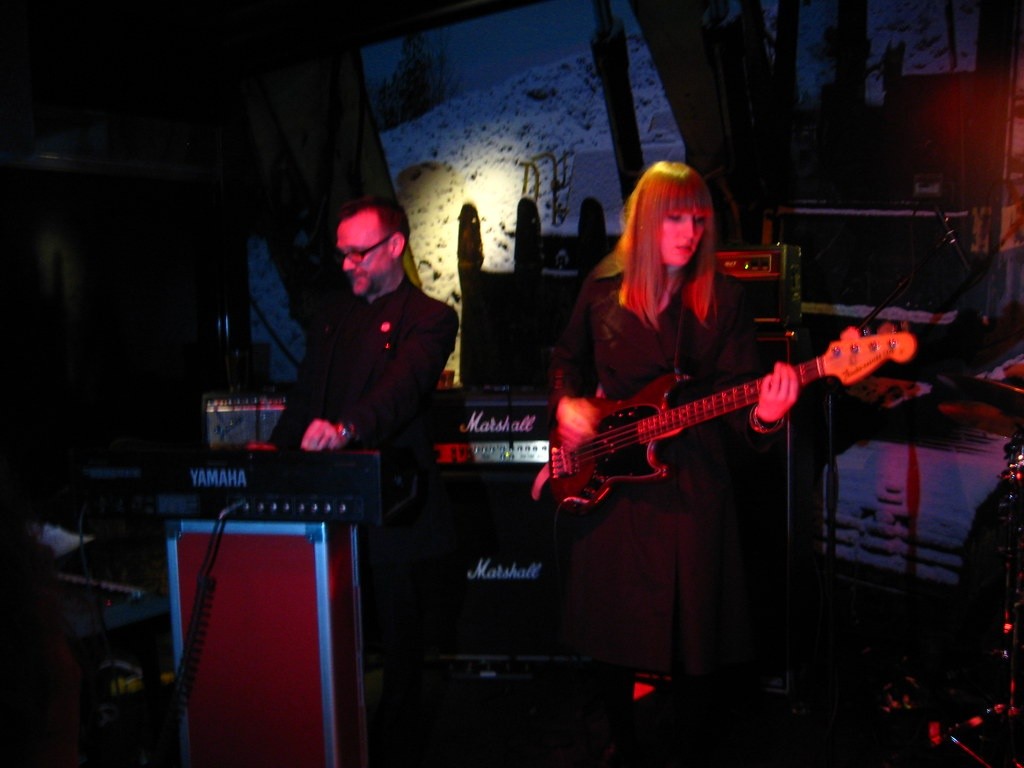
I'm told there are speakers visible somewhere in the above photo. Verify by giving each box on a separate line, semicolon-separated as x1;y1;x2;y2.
201;394;286;448
408;461;589;665
704;327;824;700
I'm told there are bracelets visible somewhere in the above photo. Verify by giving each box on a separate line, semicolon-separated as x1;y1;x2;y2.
753;407;784;432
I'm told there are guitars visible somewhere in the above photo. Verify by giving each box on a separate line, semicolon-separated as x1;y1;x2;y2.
548;315;919;513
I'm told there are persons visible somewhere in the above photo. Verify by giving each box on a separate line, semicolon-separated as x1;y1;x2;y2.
265;195;458;563
544;159;802;671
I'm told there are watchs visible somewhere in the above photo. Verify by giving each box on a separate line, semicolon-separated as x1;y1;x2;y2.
337;417;360;443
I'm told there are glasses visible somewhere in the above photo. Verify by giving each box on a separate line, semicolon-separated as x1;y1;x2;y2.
336;232;395;264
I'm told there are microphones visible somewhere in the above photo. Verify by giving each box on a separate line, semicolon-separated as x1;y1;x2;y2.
949;256;991;303
